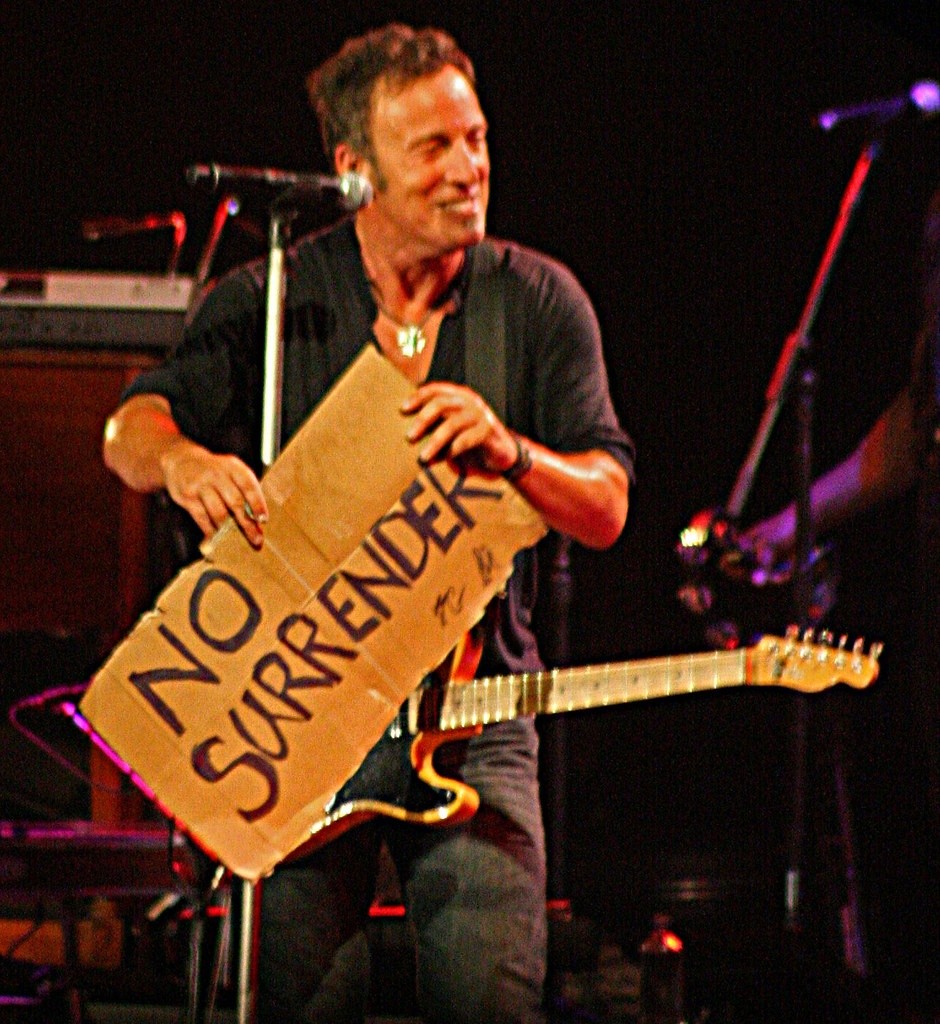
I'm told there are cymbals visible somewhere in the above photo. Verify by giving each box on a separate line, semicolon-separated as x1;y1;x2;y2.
675;503;843;649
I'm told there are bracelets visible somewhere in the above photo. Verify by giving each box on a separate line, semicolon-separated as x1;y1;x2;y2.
501;425;534;483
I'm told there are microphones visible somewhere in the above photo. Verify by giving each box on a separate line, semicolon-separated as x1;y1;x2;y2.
184;161;375;213
808;80;939;132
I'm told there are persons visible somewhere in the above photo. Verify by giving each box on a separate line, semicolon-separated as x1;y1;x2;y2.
98;21;645;1024
713;201;940;986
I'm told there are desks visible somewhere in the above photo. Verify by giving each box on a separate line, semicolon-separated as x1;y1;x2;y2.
0;347;171;663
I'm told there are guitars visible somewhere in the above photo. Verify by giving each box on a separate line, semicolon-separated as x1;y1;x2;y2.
65;625;886;884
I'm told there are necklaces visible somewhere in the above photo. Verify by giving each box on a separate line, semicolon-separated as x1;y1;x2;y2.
359;246;472;359
